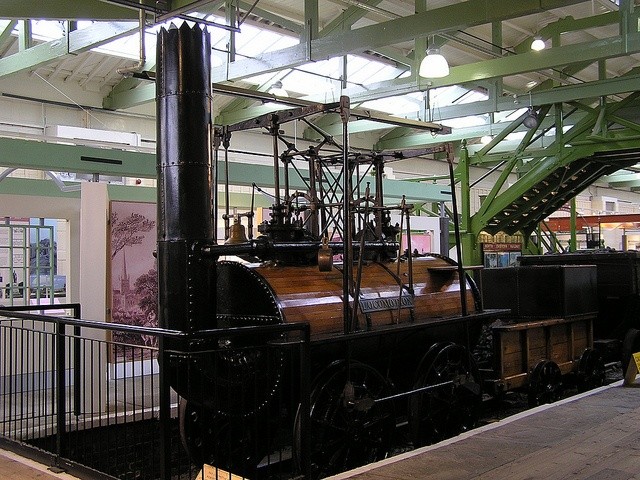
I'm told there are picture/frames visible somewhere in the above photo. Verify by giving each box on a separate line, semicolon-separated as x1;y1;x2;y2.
108;200;159;380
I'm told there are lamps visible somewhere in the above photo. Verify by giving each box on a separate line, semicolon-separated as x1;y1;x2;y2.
531;31;545;51
418;33;450;79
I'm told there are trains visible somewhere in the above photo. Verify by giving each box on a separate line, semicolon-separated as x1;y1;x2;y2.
158;95;640;479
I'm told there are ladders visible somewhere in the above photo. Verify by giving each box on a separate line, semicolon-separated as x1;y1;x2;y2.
369;77;640;278
